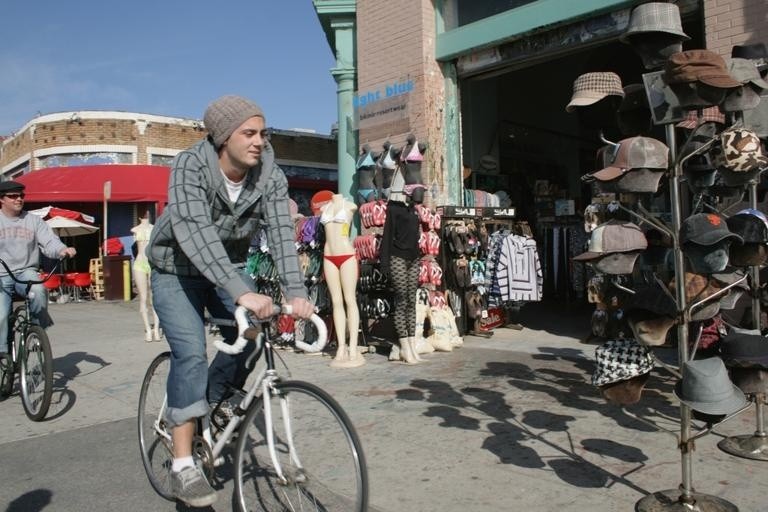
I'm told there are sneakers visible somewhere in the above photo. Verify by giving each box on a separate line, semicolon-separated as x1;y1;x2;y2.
167;464;219;508
211;402;235;443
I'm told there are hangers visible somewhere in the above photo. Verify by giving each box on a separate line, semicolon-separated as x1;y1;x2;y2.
543;219;585;229
499;221;527;239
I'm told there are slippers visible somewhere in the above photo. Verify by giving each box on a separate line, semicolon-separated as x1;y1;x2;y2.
246;216;326;345
356;199;487;321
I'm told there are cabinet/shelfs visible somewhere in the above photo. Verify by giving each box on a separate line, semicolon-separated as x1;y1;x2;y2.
101;255;130;302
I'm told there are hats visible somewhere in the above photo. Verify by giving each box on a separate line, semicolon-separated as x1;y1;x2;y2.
204;93;266;148
0;181;26;194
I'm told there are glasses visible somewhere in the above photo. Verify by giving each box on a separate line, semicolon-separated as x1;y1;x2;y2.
3;194;25;200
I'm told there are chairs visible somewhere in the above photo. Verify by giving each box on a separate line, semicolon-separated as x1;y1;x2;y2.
39;272;91;303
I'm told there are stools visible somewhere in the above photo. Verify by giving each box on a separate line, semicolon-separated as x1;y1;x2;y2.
90;258;104;300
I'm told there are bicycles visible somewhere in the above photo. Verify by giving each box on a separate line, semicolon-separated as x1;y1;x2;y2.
136;300;371;510
0;253;76;419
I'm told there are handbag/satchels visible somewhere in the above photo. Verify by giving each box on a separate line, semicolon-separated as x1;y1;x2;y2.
405;288;465;355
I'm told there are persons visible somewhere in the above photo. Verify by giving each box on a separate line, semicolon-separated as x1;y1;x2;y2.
378;186;430;365
144;93;316;508
130;218;161;343
318;194;361;361
0;180;77;372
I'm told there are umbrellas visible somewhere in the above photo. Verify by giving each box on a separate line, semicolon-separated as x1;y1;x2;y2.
44;215;100;274
26;205;96;272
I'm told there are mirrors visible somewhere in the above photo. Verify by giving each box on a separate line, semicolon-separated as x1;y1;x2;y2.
642;71;687;126
